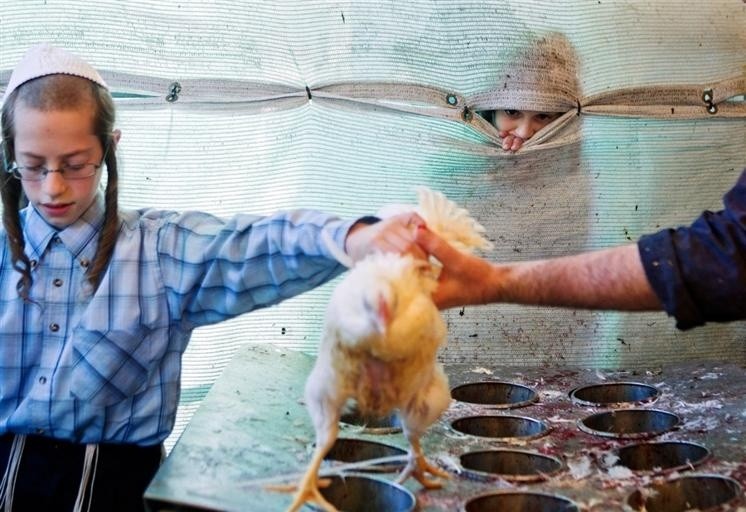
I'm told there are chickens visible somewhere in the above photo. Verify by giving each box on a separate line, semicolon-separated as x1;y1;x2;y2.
262;186;494;512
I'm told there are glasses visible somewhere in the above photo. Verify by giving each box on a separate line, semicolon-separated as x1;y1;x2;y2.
9;146;109;181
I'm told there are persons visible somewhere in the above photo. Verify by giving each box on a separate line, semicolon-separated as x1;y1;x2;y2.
411;174;746;329
430;33;604;370
1;40;433;510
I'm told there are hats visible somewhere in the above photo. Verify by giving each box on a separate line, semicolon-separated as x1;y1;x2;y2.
2;44;111;101
